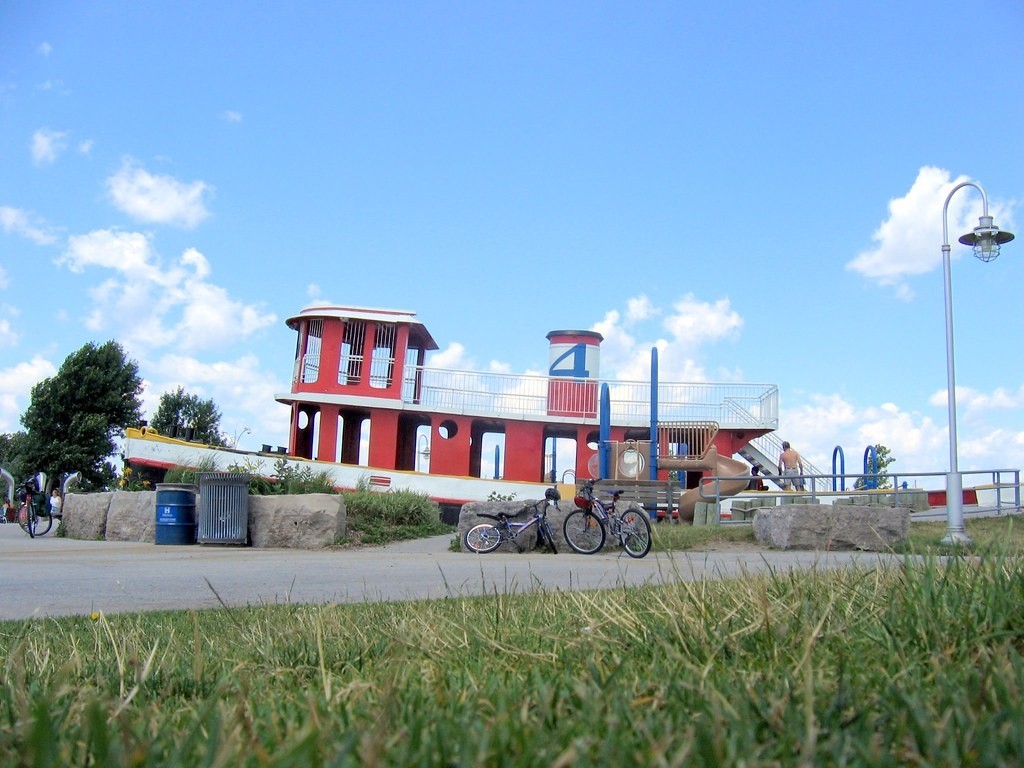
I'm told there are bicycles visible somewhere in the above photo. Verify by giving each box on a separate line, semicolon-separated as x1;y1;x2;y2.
17;472;53;539
563;478;652;559
464;485;561;554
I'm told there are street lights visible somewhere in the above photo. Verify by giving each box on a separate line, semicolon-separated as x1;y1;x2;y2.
936;182;1016;554
220;428;252;450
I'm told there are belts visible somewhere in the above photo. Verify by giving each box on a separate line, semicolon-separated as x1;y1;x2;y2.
787;468;795;469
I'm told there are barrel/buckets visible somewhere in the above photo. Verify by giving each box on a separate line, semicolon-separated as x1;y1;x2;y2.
155;483;197;545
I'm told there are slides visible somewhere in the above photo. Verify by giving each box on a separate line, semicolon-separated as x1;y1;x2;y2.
677;453;750;525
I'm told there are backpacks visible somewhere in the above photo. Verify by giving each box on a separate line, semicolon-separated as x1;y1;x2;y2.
36;491;52;516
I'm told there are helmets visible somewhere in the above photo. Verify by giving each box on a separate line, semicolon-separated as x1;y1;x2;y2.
575;497;591;510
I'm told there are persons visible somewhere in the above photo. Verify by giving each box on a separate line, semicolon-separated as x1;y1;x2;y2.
751;466;762;490
1;499;14;515
778;441;804;491
49;490;62;514
